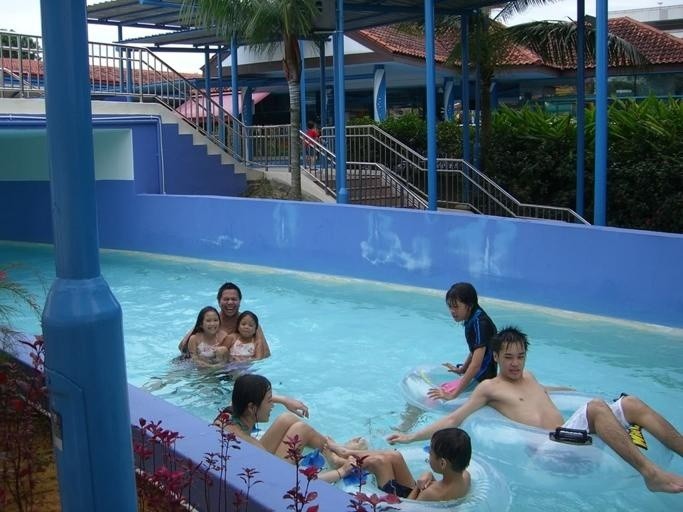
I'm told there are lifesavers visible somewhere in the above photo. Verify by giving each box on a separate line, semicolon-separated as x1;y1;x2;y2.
462;393;673;493
340;444;512;512
404;363;476;414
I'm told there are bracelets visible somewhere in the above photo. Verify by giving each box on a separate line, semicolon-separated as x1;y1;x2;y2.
336;468;342;479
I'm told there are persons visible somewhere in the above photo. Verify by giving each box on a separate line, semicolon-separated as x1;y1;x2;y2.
385;324;683;494
179;282;272;365
188;306;228;370
390;282;499;432
303;120;328;171
321;427;473;502
216;310;264;364
212;374;369;483
